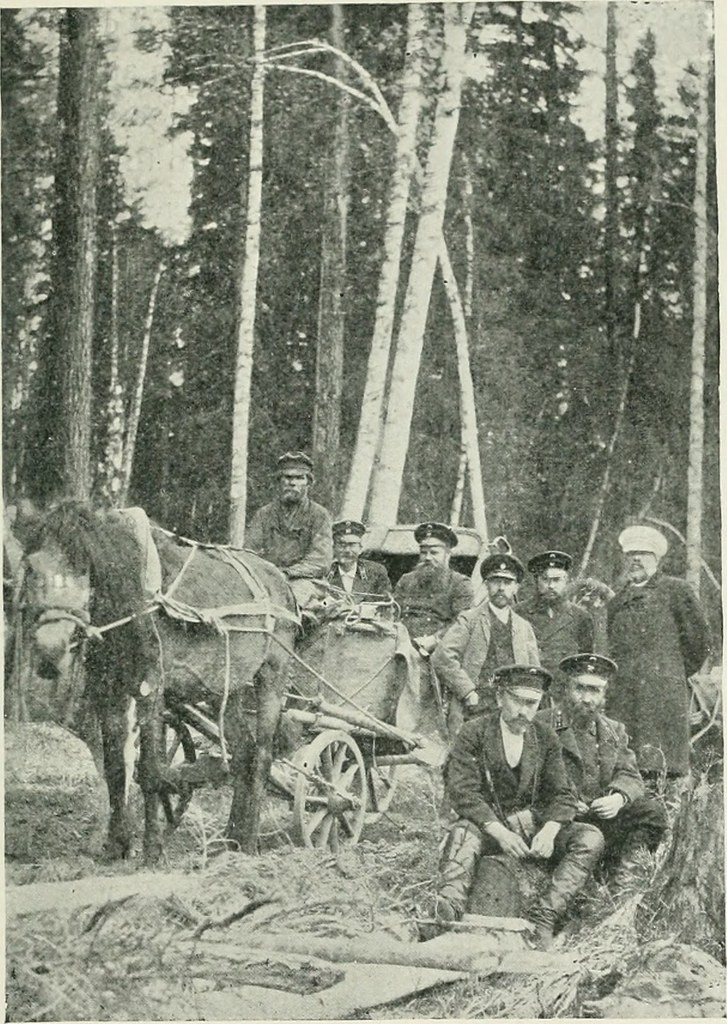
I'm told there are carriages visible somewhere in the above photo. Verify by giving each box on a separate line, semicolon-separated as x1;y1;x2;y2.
10;499;500;873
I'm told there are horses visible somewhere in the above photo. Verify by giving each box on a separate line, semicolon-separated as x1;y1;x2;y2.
10;499;301;874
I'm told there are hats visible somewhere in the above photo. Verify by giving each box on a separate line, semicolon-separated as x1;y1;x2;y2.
414;521;458;548
493;663;554;700
332;519;366;543
527;550;574;577
558;653;619;686
480;553;525;583
618;526;668;560
276;451;314;477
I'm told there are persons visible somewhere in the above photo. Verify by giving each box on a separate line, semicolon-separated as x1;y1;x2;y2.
429;554;542;727
242;451;334;580
394;523;473;654
419;665;605;941
603;527;710;775
531;653;664;890
512;551;596;678
327;521;392;603
